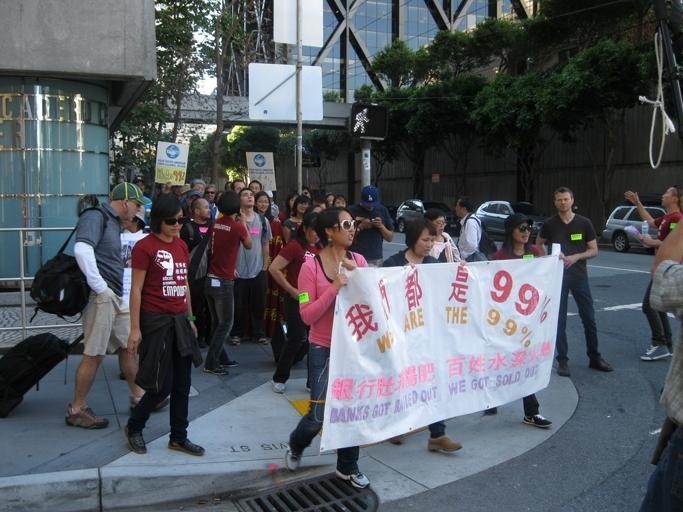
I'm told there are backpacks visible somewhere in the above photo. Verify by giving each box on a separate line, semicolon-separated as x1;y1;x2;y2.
464;215;498;262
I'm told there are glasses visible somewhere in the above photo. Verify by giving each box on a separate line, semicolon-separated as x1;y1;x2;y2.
517;223;534;233
330;219;359;233
161;216;187;225
205;190;216;194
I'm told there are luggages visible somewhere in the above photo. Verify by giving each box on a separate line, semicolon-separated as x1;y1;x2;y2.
270;287;309;365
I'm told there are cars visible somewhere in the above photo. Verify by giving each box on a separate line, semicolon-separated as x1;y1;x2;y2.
393;197;667;253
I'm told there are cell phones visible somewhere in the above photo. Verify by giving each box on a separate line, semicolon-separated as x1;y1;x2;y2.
371;218;375;222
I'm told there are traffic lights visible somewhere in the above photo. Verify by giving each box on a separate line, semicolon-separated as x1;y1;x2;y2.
350;104;386;139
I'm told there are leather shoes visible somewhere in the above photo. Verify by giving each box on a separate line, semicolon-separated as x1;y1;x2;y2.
589;353;614;372
556;359;571;377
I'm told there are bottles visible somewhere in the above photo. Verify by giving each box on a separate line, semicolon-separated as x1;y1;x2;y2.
642;219;649;234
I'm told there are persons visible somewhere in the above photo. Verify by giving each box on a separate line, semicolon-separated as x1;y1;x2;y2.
640;214;683;512
485;213;552;429
535;186;614;376
624;185;683;361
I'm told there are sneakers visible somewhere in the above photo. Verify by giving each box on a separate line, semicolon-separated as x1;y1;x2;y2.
522;414;553;429
335;465;371;490
227;333;270;347
123;424;148;455
639;340;675;361
129;394;171;412
428;434;463;452
284;443;302;474
167;436;206;456
65;402;109;429
202;360;240;376
270;378;286;394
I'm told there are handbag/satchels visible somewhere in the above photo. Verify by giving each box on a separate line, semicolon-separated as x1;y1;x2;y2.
189;220;214;281
28;208;110;317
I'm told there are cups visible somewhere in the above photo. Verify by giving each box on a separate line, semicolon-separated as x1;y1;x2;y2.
551;242;561;256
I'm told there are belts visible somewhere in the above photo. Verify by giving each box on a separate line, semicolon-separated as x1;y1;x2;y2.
205;271;226;279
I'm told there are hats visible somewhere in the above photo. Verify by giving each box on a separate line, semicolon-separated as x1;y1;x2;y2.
108;181;153;225
502;213;535;237
359;183;381;208
